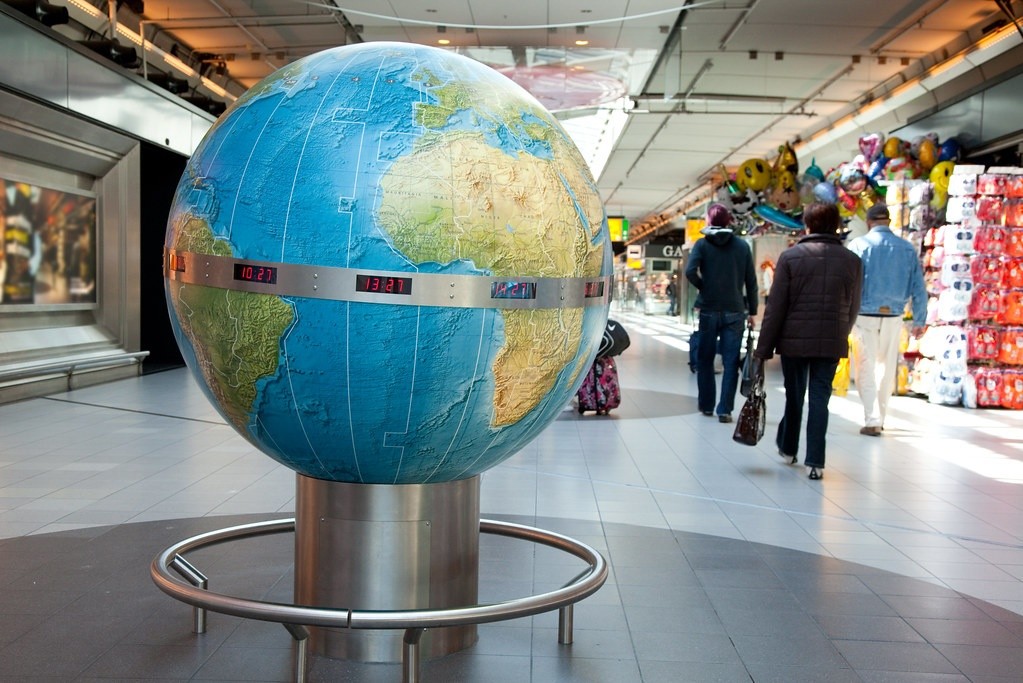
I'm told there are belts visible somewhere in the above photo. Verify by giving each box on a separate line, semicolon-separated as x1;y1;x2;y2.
858;314;898;317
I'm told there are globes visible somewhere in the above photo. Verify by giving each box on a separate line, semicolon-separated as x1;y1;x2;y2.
160;40;618;488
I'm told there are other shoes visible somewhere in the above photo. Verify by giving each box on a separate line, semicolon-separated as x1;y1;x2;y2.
698;405;713;415
779;448;797;464
718;414;732;423
806;465;823;479
860;427;884;436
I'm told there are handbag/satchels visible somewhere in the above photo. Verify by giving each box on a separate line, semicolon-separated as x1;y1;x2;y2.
740;325;755;397
733;355;766;446
596;320;630;359
831;339;852;396
666;285;672;295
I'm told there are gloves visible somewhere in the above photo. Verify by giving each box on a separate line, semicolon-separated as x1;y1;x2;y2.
757;346;773;359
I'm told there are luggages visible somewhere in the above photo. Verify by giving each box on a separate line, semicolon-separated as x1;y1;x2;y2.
688;330;701;372
576;357;621;416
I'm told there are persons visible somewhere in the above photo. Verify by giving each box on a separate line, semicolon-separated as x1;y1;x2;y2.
666;276;676;317
846;204;928;436
685;202;759;424
753;199;865;480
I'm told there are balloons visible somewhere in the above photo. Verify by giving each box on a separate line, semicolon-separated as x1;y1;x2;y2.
717;132;964;236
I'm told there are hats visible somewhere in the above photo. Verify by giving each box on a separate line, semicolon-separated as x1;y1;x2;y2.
707;205;730;226
867;205;889;220
